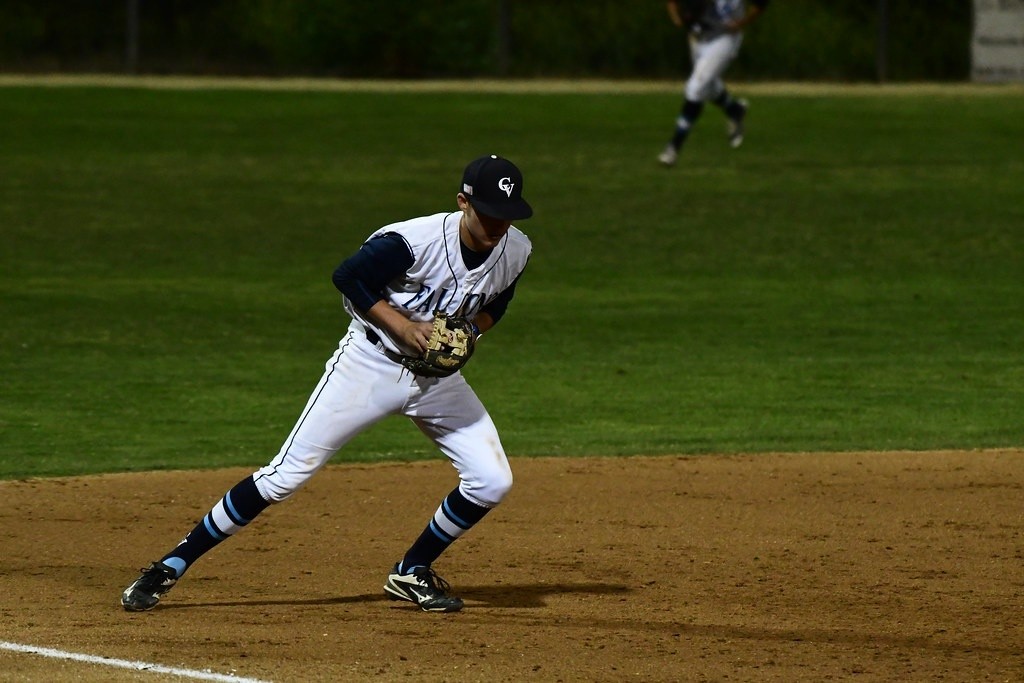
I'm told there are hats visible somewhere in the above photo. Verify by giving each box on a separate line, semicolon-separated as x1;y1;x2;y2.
460;154;533;220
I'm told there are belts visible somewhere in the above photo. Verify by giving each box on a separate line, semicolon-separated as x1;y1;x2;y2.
365;329;456;378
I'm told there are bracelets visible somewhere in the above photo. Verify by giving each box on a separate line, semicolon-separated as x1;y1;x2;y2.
472;325;480;338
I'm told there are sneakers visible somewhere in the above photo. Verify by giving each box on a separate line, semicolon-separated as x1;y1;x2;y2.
383;562;464;612
121;561;179;611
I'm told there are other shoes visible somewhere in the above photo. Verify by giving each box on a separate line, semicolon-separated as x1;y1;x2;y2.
727;98;751;149
656;142;680;166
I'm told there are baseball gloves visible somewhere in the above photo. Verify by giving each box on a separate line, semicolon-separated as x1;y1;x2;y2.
401;313;478;380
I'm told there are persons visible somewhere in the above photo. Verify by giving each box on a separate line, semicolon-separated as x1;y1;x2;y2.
120;154;533;613
656;0;769;167
